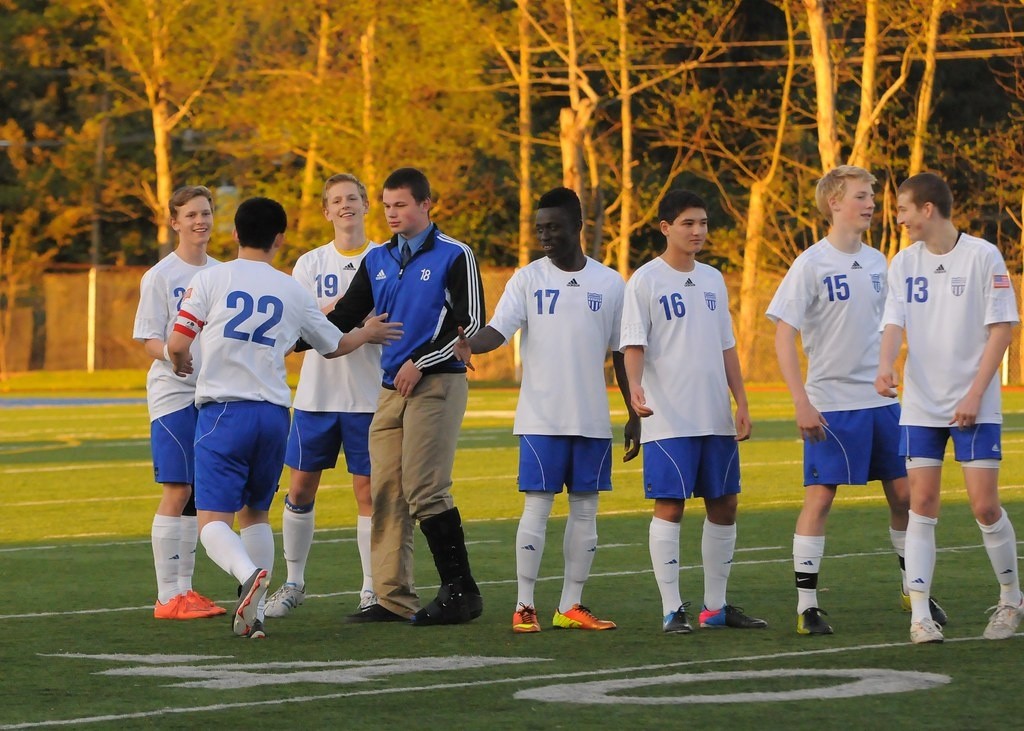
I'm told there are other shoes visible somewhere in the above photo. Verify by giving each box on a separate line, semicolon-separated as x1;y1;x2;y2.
344;603;411;623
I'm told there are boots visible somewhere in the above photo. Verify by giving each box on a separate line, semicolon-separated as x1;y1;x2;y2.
408;506;483;625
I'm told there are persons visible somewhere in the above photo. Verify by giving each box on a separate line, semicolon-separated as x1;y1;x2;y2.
765;165;948;634
452;187;642;633
875;172;1024;643
619;190;767;634
168;197;405;639
263;175;380;618
131;186;226;618
283;168;486;626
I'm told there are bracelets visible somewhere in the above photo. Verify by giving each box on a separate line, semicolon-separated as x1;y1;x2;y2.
163;344;172;361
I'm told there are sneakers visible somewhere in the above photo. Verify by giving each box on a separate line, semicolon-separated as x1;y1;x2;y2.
232;567;271;636
263;581;306;618
512;601;541;633
184;589;227;614
699;604;768;630
552;603;618;630
662;601;693;633
796;607;834;635
909;617;945;643
154;593;218;619
246;618;266;639
356;587;378;612
900;586;949;626
979;592;1024;640
